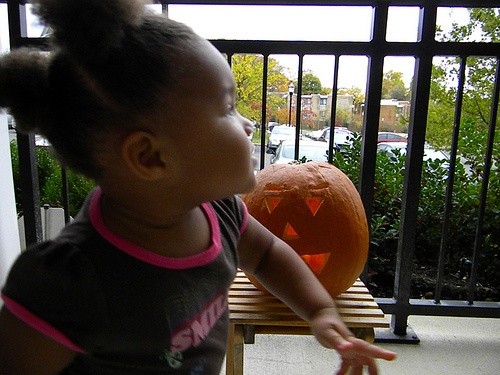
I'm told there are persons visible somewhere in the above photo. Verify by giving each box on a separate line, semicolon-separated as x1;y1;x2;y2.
0;0;396;375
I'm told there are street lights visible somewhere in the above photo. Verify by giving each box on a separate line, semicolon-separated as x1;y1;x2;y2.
288;82;296;127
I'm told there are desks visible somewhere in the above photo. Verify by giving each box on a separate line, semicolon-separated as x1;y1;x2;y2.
225;267;392;375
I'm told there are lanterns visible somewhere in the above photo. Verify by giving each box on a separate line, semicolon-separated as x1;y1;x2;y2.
240;160;370;298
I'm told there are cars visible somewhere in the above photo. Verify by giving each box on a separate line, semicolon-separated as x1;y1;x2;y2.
378;132;408;143
424;145;485;183
266;121;357;166
377;141;407;159
252;120;261;128
